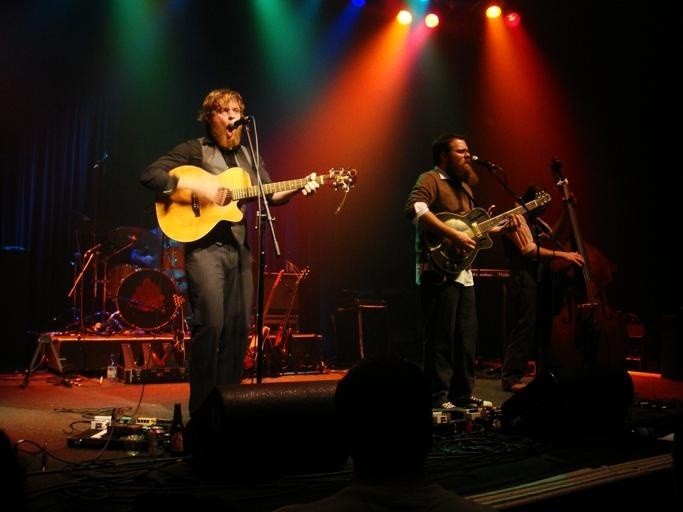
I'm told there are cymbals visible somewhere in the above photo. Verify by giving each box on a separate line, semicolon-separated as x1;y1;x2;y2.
109;227;156;255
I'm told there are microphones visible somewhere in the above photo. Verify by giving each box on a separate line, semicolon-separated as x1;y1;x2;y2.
464;152;495;168
230;116;250;129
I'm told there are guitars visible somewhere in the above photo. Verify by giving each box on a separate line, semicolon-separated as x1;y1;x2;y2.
244;266;312;373
424;190;551;276
155;165;357;243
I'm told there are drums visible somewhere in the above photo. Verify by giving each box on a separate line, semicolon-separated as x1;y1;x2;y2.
108;264;180;332
161;246;185;278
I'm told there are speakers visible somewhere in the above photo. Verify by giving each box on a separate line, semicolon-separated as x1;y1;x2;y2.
187;379;348;484
500;365;634;447
289;334;324;373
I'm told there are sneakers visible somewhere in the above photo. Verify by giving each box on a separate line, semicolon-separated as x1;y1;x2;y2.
447;393;492;409
432;399;457;409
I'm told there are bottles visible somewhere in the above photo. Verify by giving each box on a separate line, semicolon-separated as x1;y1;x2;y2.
107;354;116;381
170;404;184;457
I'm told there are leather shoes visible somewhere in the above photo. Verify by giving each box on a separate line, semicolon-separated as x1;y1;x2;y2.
510;383;528;392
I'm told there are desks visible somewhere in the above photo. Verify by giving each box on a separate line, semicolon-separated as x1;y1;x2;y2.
20;334;324;388
330;304;384;361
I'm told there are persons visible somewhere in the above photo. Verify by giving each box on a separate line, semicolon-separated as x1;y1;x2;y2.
139;86;321;419
402;133;523;410
500;183;586;393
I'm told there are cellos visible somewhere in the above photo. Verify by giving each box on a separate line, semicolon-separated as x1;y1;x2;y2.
547;161;627;383
